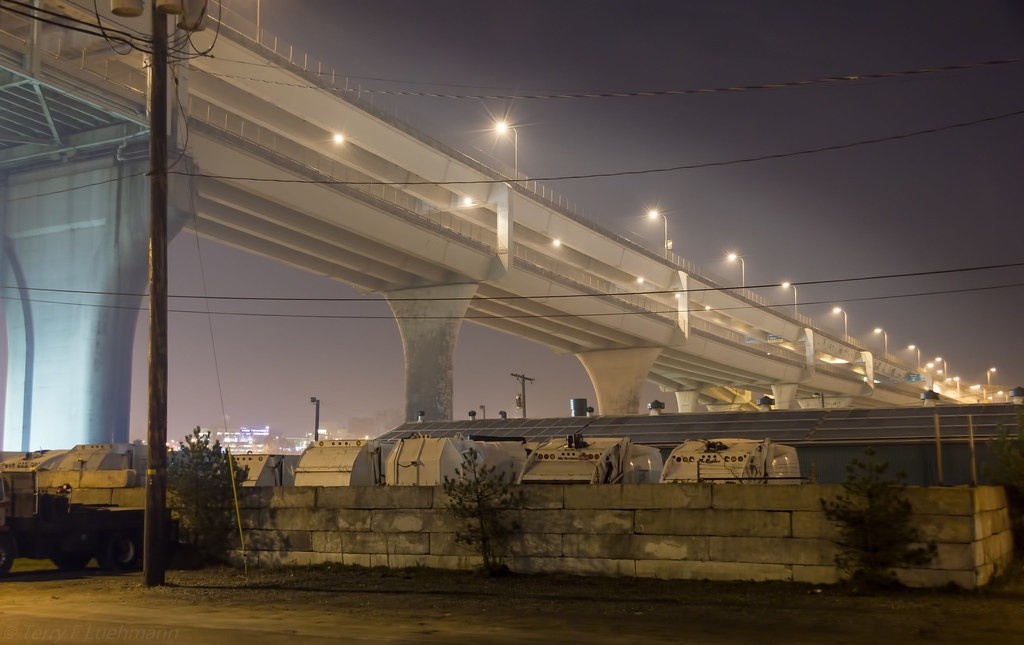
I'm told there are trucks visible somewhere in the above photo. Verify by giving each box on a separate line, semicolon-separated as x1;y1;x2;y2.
0;466;176;577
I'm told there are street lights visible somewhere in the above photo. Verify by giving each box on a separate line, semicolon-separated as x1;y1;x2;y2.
497;122;517;182
783;282;797;319
480;404;486;419
986;367;996;384
649;209;669;259
934;356;947;382
812;392;824;409
923;360;933;371
834;308;848;340
875;328;888;359
909;345;920;371
954;376;960;393
728;254;745;297
310;396;321;441
